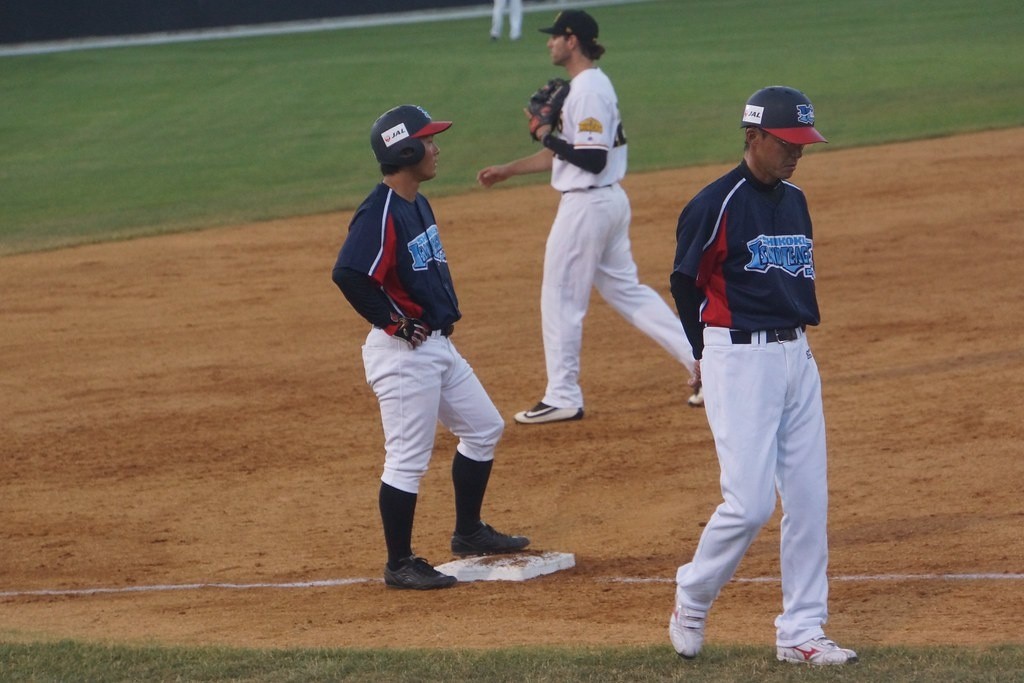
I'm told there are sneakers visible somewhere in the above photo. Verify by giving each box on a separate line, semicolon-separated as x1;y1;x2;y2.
669;594;706;659
384;554;458;590
688;386;704;406
514;402;583;424
777;635;858;665
451;520;530;555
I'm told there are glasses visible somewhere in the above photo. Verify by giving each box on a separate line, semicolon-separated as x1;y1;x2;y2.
763;132;805;150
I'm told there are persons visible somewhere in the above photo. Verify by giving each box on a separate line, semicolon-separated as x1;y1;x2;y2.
669;86;859;664
490;0;523;40
476;8;704;425
330;106;530;590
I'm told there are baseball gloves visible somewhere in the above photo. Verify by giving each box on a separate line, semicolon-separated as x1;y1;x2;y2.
522;78;571;138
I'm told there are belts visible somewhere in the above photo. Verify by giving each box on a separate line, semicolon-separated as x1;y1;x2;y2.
427;325;454;336
730;325;806;344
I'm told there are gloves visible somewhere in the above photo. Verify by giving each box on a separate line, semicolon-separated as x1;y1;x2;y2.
393;318;430;350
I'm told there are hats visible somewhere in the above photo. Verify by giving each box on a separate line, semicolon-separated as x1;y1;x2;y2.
740;86;829;144
537;10;599;41
370;105;452;165
